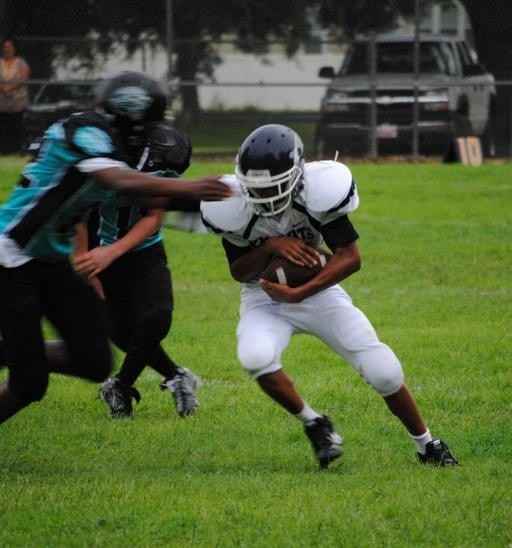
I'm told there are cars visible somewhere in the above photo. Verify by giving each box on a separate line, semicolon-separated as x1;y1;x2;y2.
23;75;110;158
312;32;497;162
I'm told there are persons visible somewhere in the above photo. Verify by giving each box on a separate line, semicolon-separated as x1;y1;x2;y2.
200;122;460;471
71;122;200;419
2;71;236;425
1;38;30;155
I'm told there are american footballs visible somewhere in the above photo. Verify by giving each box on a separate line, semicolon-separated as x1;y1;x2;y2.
262;247;333;288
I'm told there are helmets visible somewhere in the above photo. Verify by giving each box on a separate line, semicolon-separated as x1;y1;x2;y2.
138;124;191;175
235;123;303;219
96;71;166;142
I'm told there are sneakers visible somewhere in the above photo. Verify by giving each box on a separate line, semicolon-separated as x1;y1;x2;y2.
160;367;201;418
416;438;460;469
306;420;344;469
99;374;141;420
